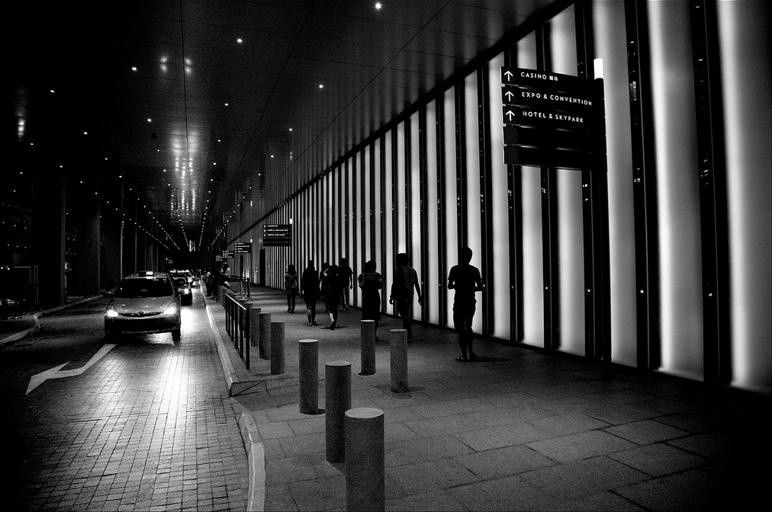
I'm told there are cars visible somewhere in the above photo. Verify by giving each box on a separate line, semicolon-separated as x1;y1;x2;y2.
104;269;196;341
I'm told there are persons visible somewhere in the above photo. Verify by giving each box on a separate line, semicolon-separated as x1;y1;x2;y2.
390;253;423;341
447;247;482;361
192;264;231;297
284;257;384;338
